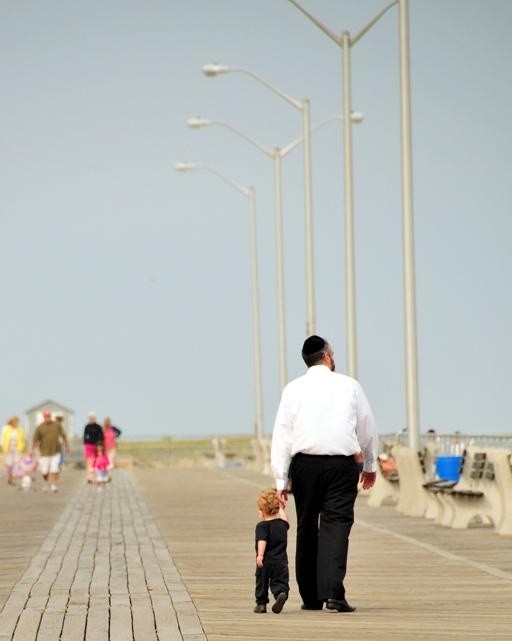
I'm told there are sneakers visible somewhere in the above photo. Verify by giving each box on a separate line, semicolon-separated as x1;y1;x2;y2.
272;593;286;614
253;605;266;613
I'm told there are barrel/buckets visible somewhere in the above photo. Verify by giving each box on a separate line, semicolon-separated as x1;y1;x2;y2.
434;454;464;483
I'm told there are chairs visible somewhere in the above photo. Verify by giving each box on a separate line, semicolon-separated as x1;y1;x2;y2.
360;442;512;536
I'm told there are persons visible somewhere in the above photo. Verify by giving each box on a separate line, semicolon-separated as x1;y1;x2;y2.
253;490;290;613
1;408;121;494
271;334;379;613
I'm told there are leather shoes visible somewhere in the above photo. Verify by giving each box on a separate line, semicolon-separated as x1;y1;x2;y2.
326;599;356;612
300;603;323;610
